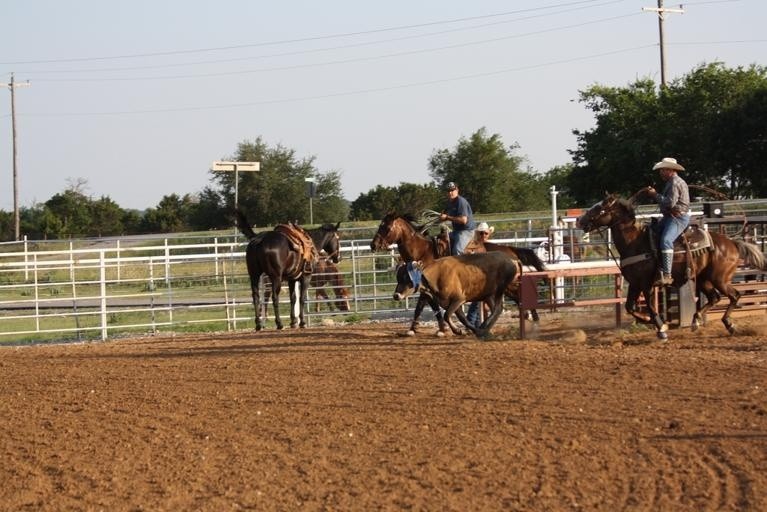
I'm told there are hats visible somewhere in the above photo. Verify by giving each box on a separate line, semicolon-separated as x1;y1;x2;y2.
653;158;684;171
446;182;456;192
474;223;494;234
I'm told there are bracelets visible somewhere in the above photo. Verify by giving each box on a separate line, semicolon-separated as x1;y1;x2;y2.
446;215;448;220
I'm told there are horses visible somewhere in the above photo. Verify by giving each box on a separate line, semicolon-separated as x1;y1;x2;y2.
236;197;342;333
531;236;606;285
579;190;766;340
370;209;548;337
262;253;350;318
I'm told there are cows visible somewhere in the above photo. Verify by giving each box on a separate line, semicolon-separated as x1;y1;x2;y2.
393;250;522;338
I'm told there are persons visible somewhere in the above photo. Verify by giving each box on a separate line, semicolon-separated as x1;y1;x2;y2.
465;222;495;333
645;157;691;288
437;181;475;256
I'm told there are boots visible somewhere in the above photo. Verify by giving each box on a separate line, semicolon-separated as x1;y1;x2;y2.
654;249;673;285
